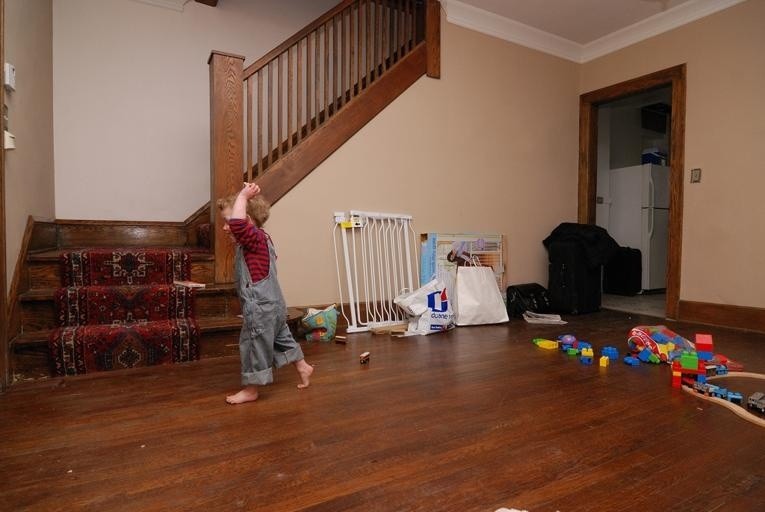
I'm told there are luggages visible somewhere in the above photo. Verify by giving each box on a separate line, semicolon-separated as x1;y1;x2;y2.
549;241;601;314
603;246;641;296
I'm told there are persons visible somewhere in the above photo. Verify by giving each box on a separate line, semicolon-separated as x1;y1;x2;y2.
216;182;314;405
446;239;470;273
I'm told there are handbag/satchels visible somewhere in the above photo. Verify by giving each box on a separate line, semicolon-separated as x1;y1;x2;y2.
505;283;553;319
394;275;455;335
543;222;619;275
302;304;340;343
454;255;510;327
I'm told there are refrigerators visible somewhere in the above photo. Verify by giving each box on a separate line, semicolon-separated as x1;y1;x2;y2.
610;164;669;290
